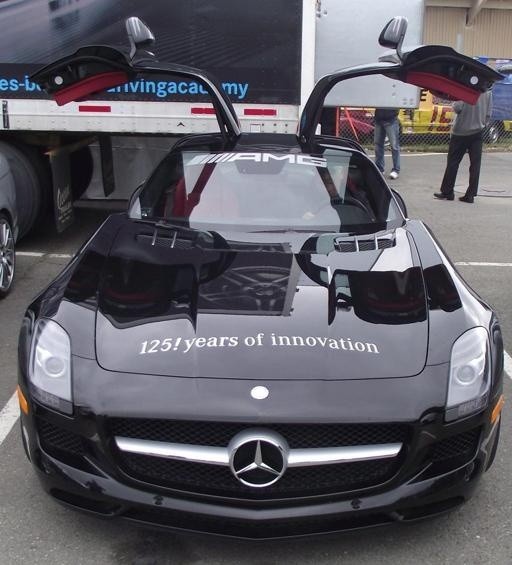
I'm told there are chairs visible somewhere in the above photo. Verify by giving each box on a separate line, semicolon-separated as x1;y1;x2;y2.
165;168;240;221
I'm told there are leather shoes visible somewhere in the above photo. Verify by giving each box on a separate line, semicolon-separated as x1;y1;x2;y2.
434;193;454;200
459;196;474;203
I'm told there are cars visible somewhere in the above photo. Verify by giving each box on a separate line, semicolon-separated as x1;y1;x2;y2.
0;151;19;298
432;60;511;144
15;16;506;542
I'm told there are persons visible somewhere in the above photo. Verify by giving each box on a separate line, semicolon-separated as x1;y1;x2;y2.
431;88;493;205
372;108;401;179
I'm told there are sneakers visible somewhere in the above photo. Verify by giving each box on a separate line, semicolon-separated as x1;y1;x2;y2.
389;172;398;178
